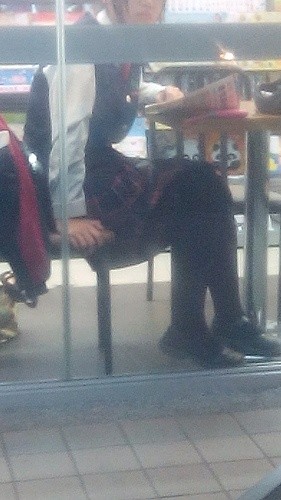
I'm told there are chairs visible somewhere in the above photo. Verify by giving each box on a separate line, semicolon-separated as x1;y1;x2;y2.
2;116;173;377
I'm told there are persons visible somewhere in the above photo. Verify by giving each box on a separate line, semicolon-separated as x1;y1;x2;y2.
22;0;281;367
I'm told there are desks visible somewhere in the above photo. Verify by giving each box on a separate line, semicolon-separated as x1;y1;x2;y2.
140;98;281;336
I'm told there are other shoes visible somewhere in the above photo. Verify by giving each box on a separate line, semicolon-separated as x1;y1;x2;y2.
160;325;243;369
211;310;281;358
